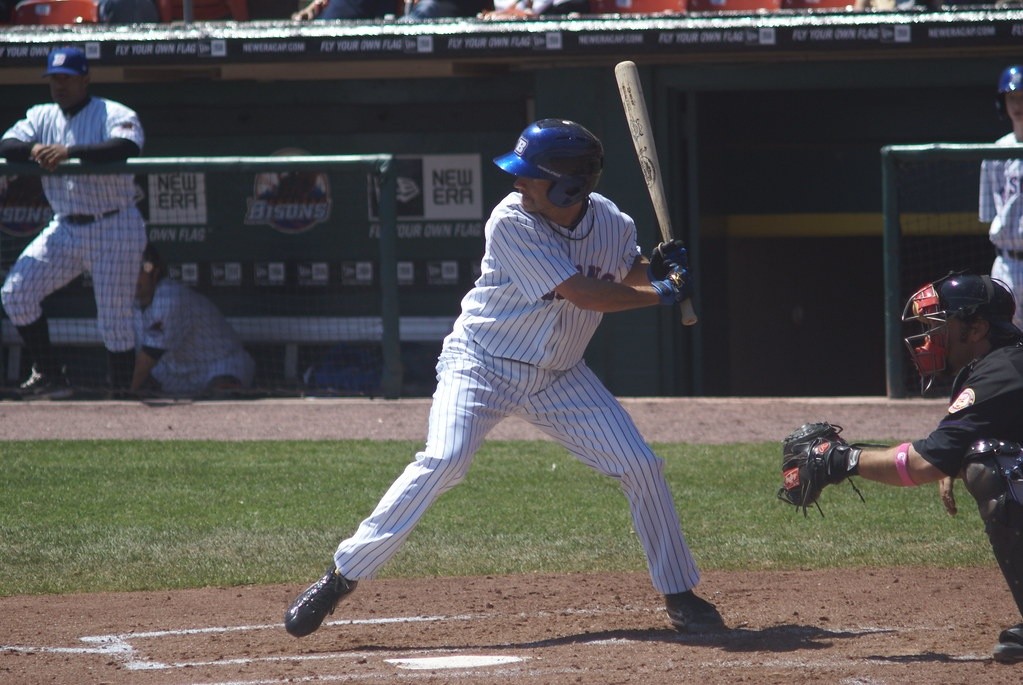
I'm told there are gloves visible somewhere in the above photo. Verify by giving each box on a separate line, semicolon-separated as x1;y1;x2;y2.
649;239;689;282
650;265;692;307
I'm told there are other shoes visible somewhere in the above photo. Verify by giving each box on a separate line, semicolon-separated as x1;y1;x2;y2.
994;622;1023;663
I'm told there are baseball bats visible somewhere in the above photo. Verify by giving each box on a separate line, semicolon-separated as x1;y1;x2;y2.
614;59;699;327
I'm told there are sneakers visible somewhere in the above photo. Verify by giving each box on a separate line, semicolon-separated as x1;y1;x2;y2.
664;590;725;633
284;566;358;638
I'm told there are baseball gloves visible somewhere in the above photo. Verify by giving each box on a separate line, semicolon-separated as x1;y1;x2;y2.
781;421;853;506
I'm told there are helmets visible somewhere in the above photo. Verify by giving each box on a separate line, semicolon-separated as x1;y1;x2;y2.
492;118;605;207
899;271;1021;335
41;46;90;76
997;64;1023;95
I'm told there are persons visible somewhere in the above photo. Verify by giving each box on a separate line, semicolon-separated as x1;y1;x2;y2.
782;273;1023;661
286;118;730;639
130;241;255;400
291;0;590;22
0;46;148;401
978;64;1023;333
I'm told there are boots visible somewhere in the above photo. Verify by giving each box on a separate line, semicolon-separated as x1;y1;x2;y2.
16;315;63;392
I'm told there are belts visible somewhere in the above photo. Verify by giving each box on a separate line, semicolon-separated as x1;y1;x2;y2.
58;209;119;226
994;248;1023;260
